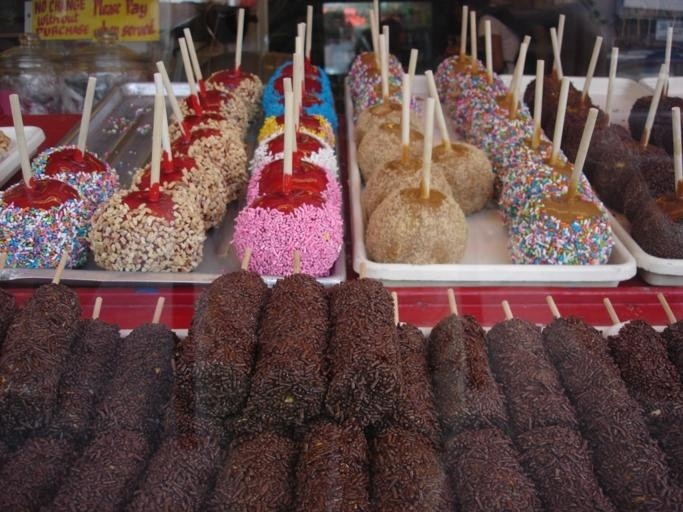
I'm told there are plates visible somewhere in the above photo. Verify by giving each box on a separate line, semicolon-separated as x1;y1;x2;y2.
501;77;683;288
0;125;45;189
17;82;346;289
344;76;637;287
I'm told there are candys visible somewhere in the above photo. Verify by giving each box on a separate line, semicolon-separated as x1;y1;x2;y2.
0;141;119;269
91;69;682;275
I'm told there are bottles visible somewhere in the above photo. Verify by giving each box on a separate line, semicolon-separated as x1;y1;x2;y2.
0;32;57;114
60;29;146;110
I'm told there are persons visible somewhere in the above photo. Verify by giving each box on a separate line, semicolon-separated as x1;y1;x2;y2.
455;0;522;76
